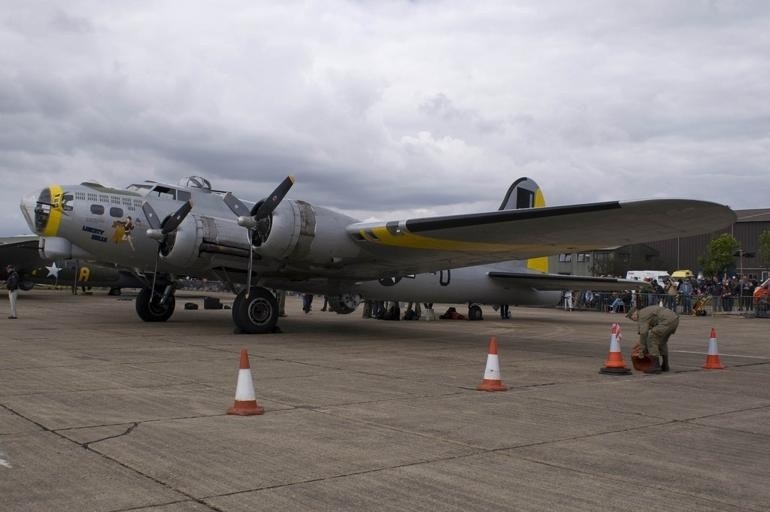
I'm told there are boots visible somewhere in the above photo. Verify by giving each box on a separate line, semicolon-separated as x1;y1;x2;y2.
644;354;669;374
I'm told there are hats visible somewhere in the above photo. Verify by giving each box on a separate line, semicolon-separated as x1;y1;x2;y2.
625;305;637;318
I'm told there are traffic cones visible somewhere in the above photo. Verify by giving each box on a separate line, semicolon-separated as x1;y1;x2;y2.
227;347;265;415
598;323;633;376
702;328;727;368
476;337;509;391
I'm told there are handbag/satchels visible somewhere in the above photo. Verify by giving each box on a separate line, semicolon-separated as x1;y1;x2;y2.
631;344;652;371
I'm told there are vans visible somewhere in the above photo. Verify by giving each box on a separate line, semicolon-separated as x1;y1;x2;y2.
626;271;672;307
753;277;770;310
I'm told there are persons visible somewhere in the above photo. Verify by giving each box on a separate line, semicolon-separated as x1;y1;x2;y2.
625;305;680;373
117;216;136;252
6;264;19;319
302;293;314;313
501;304;510;319
321;295;334;312
564;272;759;315
